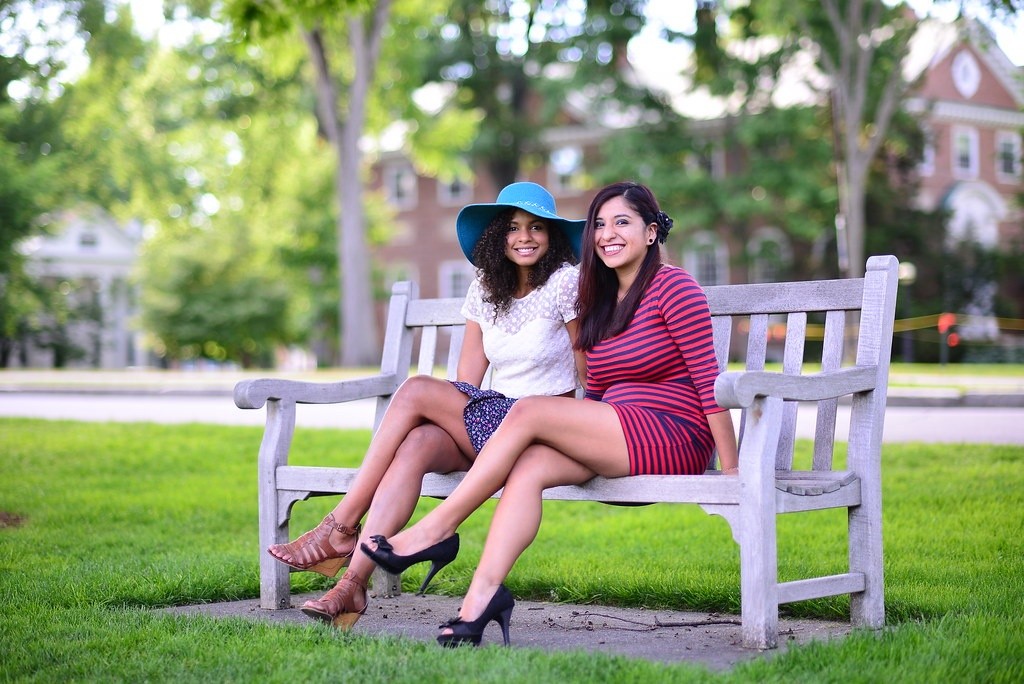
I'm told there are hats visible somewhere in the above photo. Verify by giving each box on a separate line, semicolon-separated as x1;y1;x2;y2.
456;181;587;267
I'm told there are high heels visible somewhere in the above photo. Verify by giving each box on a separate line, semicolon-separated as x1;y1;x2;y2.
300;569;368;634
266;512;360;577
436;581;514;647
361;534;460;592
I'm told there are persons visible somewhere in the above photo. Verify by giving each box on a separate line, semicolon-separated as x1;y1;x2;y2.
359;181;738;649
268;183;587;639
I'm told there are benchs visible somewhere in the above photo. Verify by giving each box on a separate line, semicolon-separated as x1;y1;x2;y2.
232;256;900;650
